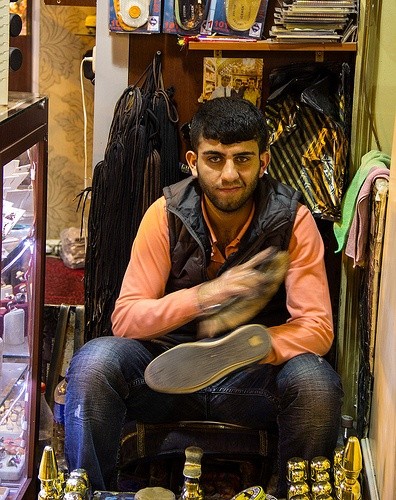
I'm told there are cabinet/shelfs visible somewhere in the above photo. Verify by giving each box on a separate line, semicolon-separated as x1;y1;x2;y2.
1;92;48;500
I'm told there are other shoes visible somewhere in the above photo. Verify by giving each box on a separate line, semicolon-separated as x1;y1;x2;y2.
142;323;275;396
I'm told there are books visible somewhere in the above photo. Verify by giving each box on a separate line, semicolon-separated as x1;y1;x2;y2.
266;0;360;44
109;0;269;40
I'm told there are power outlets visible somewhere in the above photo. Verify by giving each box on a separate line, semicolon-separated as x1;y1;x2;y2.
92;46;96;73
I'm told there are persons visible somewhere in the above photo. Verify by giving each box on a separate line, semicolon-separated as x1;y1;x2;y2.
209;77;261;109
65;98;344;500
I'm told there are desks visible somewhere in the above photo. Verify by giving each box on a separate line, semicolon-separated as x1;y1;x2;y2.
43;255;84;407
